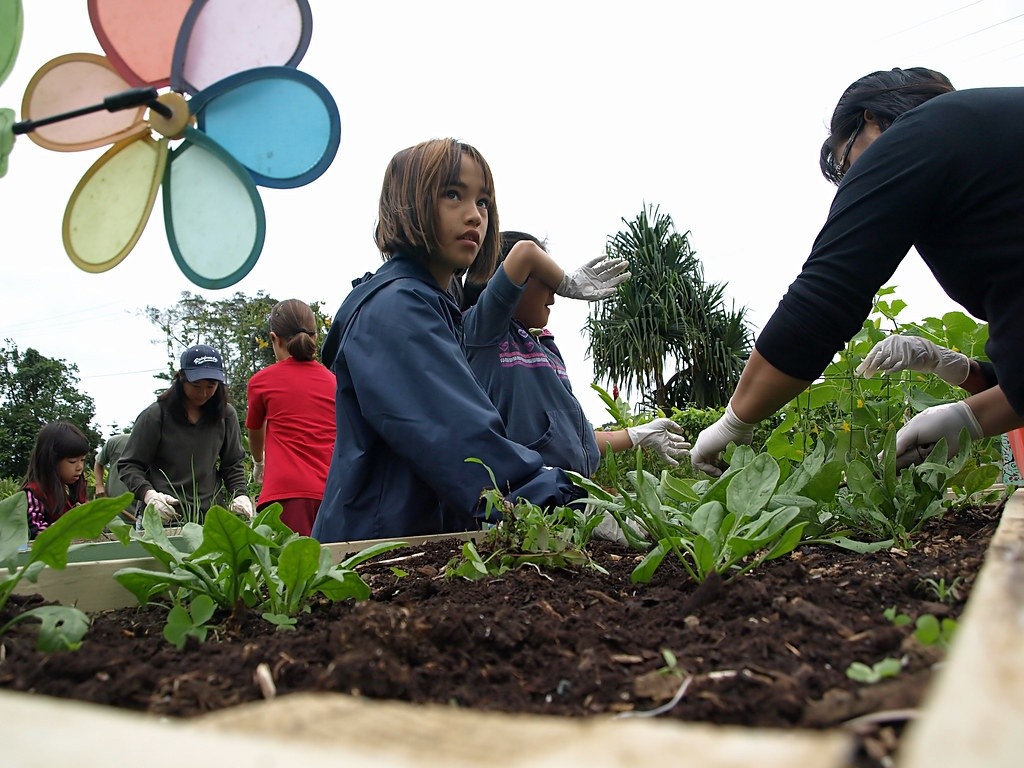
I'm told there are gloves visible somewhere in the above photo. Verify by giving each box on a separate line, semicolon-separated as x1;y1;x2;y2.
689;397;757;478
251;454;265;484
854;333;970;387
590;505;628;549
143;490;181;524
228;495;253;521
553;254;632;300
876;399;985;472
625;418;692;468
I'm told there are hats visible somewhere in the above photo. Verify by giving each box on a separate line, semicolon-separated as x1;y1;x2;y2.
182;344;224;382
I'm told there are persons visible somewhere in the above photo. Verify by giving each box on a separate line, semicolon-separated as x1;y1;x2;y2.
117;345;253;530
93;435;142;533
855;334;1024;468
310;137;648;545
690;66;1023;476
245;299;337;536
466;230;691;477
19;423;91;540
